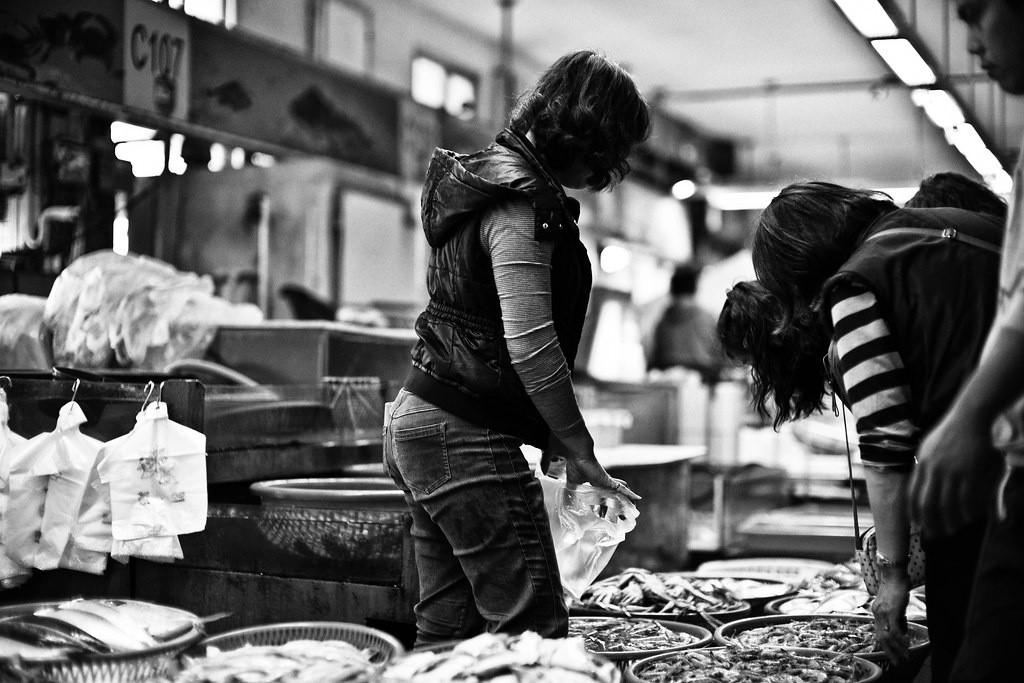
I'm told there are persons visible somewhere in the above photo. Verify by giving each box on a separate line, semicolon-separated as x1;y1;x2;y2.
382;49;656;655
641;262;728;387
910;0;1023;683
717;170;1010;675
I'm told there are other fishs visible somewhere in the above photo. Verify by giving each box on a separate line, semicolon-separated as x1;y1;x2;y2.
1;593;619;683
726;562;928;620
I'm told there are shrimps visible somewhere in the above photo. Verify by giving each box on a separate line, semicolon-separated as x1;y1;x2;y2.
565;612;932;683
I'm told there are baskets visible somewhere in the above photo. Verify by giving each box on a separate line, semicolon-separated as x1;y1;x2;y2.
192;620;405;683
625;644;883;683
668;569;794;612
714;611;932;683
566;587;753;627
694;557;842;585
1;598;202;683
376;634;623;683
762;594;929;621
563;614;712;660
248;472;410;566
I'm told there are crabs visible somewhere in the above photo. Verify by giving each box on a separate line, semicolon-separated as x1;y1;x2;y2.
579;565;741;631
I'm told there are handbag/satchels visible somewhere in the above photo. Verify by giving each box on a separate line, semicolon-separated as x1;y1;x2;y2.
853;518;926;596
